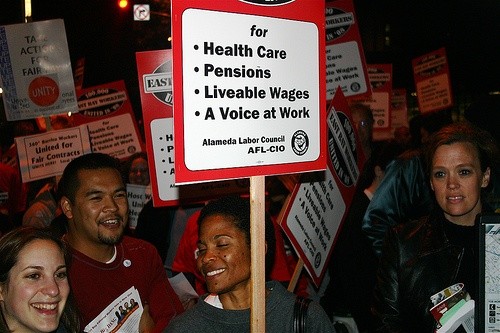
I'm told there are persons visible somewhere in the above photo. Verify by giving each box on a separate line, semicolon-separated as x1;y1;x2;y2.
130;298;138;308
268;103;500;333
0;122;252;333
162;196;334;333
112;311;122;324
119;305;127;316
124;302;132;312
